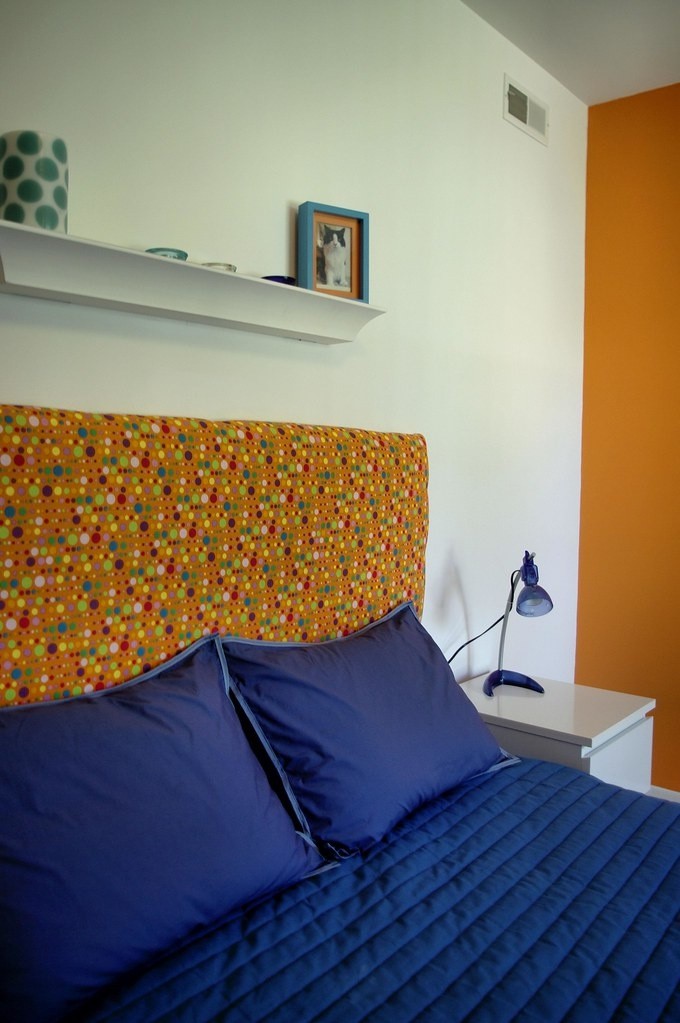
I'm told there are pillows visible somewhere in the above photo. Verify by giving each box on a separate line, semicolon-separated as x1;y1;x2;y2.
219;601;522;861
0;629;340;1023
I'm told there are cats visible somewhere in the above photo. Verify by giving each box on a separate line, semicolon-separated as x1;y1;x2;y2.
316;222;352;289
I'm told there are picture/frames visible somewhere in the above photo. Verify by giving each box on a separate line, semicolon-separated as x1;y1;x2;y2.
298;201;371;305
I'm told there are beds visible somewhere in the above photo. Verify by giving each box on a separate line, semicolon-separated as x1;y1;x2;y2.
0;402;680;1023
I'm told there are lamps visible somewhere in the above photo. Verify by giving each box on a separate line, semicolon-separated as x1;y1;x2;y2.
481;551;554;697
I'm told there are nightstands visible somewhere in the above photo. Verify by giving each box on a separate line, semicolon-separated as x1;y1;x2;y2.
456;671;656;795
